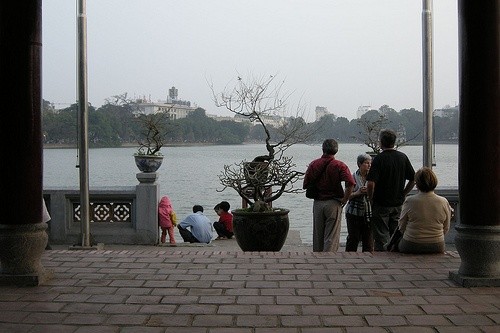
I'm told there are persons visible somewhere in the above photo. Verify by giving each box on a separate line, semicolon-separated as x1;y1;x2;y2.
366;129;416;251
398;168;451;253
178;205;213;244
303;138;355;251
158;196;177;244
214;201;234;240
220;201;233;216
43;197;53;250
345;153;374;252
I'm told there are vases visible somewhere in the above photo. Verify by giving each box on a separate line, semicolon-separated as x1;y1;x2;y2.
134;154;163;173
232;209;290;252
244;162;269;184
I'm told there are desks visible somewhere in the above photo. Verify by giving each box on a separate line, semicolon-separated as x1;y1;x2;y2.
242;184;273;209
